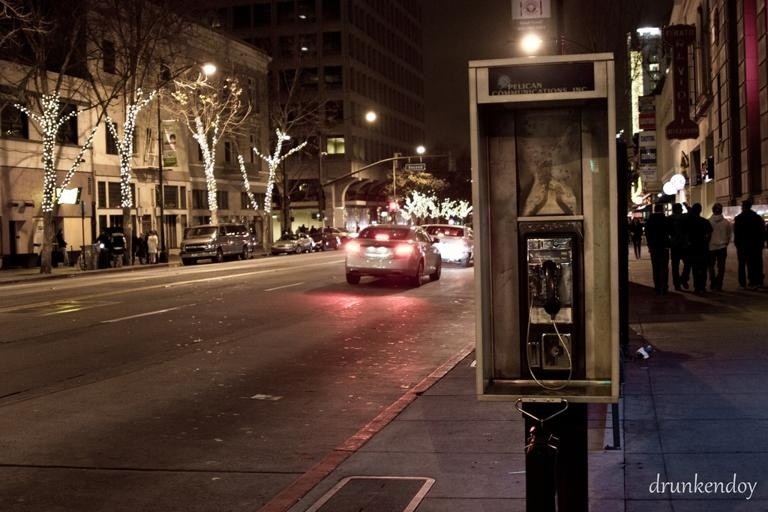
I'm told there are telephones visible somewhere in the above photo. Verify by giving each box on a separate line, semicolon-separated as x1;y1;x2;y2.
511;220;593;393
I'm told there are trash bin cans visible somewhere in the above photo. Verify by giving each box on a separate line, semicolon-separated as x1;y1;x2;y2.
80;244;100;270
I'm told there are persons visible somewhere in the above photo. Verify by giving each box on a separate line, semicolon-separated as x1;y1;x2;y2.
627;200;768;296
57;229;70;266
94;226;158;268
298;224;331;237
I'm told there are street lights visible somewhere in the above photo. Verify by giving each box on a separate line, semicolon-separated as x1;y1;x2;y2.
317;110;376;221
157;62;217;253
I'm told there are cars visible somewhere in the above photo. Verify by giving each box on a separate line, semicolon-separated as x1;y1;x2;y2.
271;226;353;254
345;224;474;287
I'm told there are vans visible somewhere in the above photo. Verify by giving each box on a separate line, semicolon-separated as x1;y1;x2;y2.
180;224;254;265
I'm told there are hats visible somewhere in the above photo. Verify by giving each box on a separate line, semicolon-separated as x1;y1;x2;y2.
713;203;722;212
